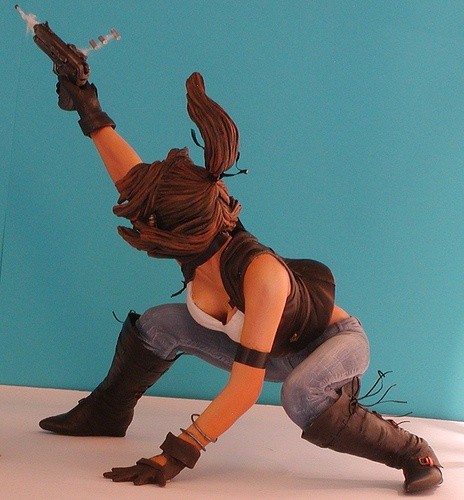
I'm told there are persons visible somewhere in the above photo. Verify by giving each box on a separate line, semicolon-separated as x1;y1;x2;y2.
39;71;443;493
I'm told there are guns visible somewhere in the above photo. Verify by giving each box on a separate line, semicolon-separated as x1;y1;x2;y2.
15;4;89;110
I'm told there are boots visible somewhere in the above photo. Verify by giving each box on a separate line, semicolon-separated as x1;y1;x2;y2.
301;369;443;491
40;310;183;437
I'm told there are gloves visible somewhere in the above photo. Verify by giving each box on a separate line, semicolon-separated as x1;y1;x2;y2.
103;431;200;487
55;76;116;138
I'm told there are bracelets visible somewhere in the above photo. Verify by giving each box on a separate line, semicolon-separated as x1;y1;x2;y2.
190;413;218;443
178;428;207;451
